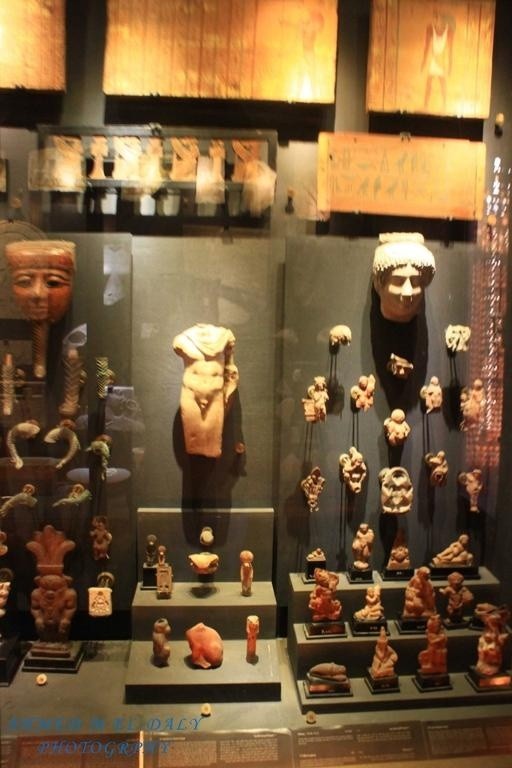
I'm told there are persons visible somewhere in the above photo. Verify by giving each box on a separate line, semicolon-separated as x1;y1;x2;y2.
420;12;454;110
7;230;509;678
